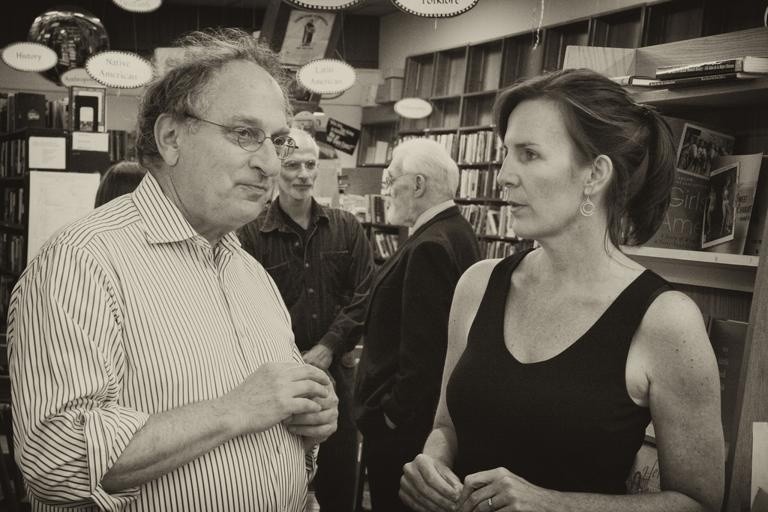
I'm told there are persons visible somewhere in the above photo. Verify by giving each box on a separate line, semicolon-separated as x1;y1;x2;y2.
678;135;733;241
234;125;378;512
398;68;727;510
351;138;482;511
92;160;146;209
4;32;341;512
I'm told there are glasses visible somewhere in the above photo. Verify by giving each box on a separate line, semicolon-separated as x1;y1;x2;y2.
184;112;299;161
380;168;408;191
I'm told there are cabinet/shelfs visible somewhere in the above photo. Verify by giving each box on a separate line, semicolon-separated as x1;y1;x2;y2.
0;128;136;511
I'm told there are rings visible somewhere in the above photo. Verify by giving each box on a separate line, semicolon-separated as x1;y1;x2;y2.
487;497;494;507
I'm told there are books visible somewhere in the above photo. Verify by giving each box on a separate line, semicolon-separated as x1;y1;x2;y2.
338;125;541;270
1;82;140;401
607;55;768;87
